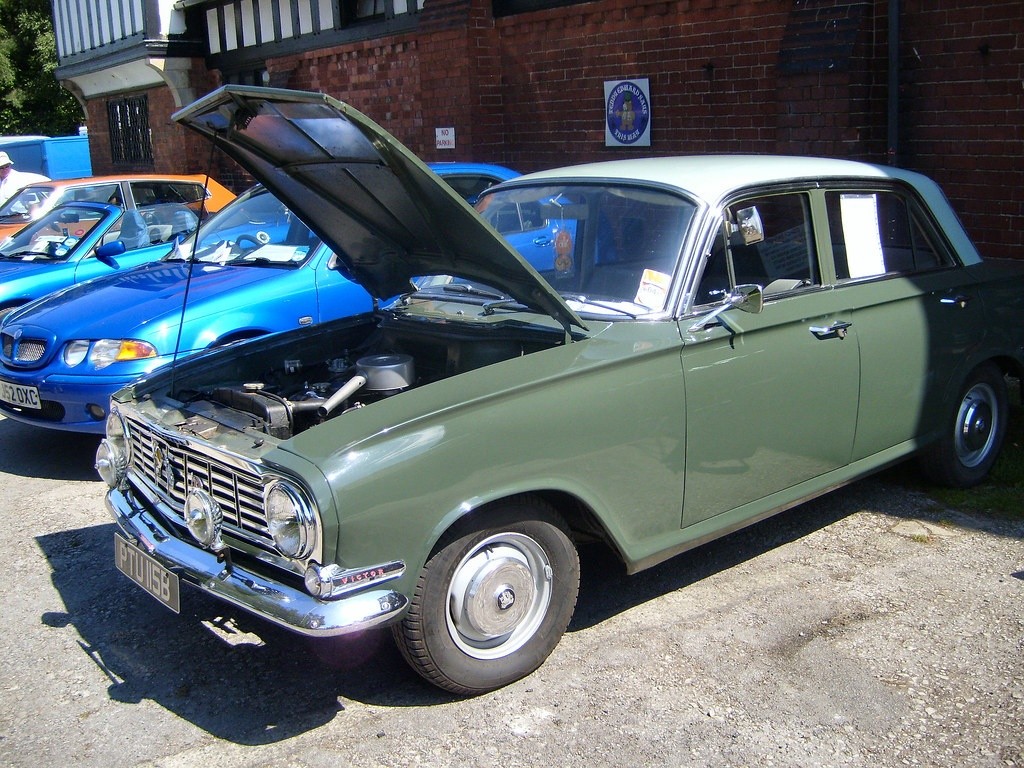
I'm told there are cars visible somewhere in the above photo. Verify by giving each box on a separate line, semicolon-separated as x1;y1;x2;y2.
94;83;1024;697
0;163;614;439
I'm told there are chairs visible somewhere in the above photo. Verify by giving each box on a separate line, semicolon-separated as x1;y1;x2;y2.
171;210;194;241
117;209;150;250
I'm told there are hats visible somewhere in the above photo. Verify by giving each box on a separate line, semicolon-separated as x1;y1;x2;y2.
0;151;14;166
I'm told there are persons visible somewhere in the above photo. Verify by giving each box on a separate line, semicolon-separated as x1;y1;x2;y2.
0;151;51;214
